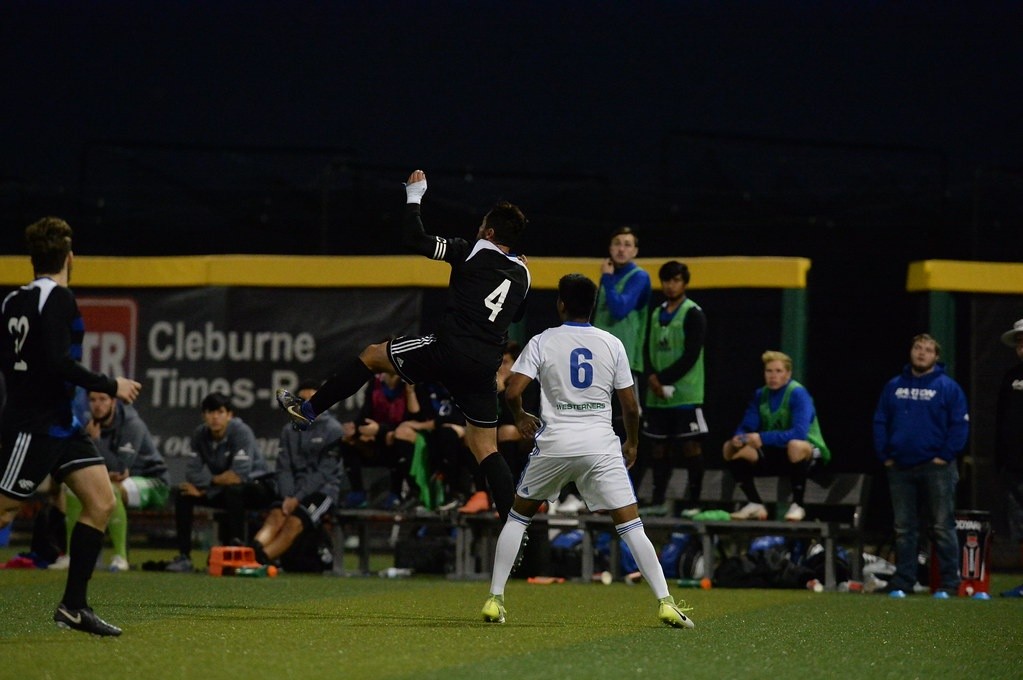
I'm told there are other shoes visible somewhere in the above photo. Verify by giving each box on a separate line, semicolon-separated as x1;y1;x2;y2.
784;503;805;521
731;503;768;521
890;589;906;598
681;507;700;518
167;556;193;573
640;504;668;517
934;591;950;599
110;556;130;573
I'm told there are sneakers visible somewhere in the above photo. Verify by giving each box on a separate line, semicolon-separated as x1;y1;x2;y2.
48;555;70;570
378;565;416;579
658;600;694;629
509;534;530;577
276;389;311;432
54;604;123;636
482;598;506;623
457;491;490;513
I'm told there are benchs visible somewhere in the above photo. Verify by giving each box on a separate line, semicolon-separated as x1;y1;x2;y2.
73;452;919;589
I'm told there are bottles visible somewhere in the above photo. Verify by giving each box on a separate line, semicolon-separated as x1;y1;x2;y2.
377;568;411;581
676;578;711;588
235;565;276;578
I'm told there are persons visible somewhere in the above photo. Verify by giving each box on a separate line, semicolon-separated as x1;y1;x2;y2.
162;390;258;575
870;332;968;596
991;320;1023;598
0;216;141;636
480;272;697;631
730;350;832;521
341;342;523;514
640;261;711;515
276;168;530;579
232;379;346;564
20;388;171;572
595;227;653;409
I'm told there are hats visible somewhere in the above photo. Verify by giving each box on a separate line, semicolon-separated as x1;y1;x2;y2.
1001;320;1023;348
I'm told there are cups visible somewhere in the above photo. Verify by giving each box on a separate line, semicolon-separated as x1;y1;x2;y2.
807;581;823;592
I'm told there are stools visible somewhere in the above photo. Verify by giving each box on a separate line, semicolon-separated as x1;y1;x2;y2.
209;545;262;576
